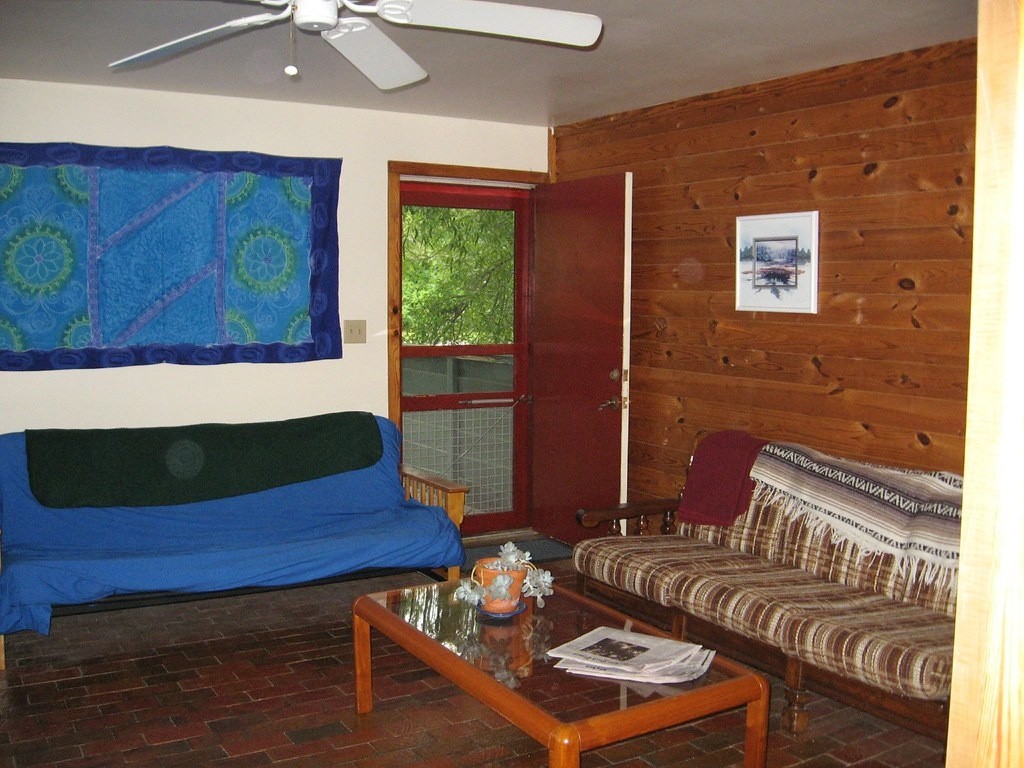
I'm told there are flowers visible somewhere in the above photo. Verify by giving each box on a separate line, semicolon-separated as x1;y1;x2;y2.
454;540;554;609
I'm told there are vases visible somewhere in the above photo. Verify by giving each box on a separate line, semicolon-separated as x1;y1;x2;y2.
474;557;527;614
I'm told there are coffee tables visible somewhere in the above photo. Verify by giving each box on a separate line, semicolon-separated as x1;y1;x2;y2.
351;581;770;768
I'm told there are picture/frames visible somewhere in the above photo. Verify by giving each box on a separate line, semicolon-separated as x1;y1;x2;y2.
734;211;818;315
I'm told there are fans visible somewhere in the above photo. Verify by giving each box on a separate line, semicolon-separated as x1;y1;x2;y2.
108;0;603;92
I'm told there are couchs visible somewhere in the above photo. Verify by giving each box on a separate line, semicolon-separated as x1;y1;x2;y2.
0;412;470;672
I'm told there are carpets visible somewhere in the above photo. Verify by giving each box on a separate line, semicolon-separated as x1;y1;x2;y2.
462;536;572;574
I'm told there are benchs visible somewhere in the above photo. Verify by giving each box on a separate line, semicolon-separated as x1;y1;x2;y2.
571;429;963;744
